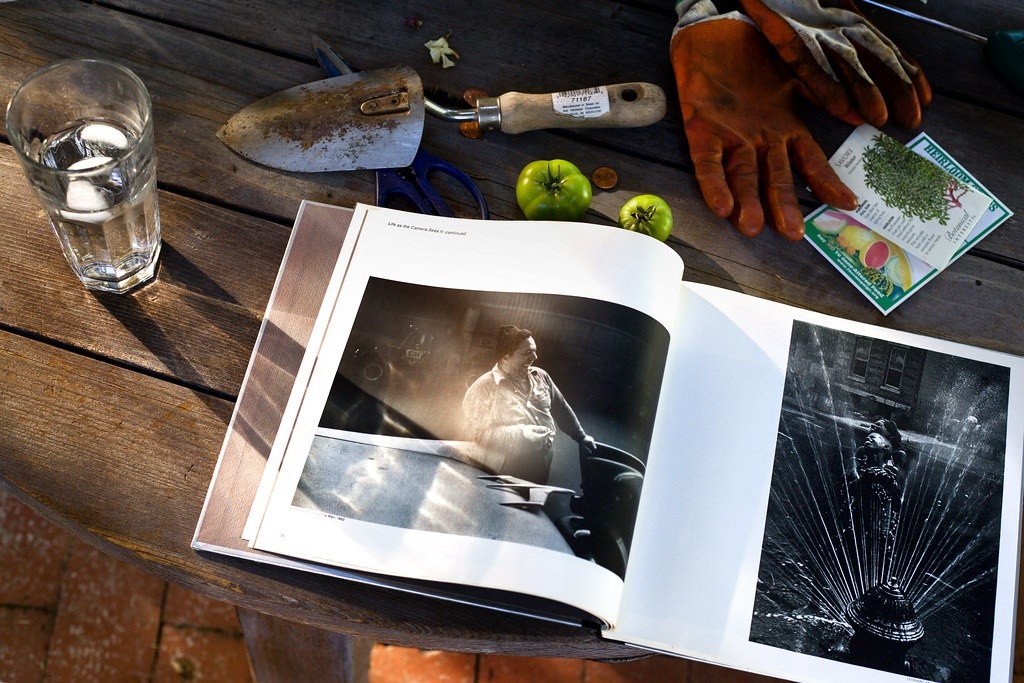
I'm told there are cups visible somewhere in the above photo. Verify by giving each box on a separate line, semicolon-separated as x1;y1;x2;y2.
6;57;163;294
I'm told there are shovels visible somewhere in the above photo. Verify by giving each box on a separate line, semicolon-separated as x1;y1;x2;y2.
214;62;667;173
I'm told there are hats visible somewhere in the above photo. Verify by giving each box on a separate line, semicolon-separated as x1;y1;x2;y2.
495;325;532;358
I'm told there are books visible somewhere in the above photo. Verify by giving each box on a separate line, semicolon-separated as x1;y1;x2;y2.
192;199;1023;683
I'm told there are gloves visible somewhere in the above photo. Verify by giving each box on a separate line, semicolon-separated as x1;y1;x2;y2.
671;0;859;240
743;0;932;128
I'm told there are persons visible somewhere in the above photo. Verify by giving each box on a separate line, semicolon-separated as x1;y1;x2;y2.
461;321;596;486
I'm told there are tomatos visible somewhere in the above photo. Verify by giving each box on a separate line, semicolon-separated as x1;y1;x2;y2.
618;195;673;242
516;158;592;223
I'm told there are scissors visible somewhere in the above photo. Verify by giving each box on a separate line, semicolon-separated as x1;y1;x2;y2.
309;32;489;223
